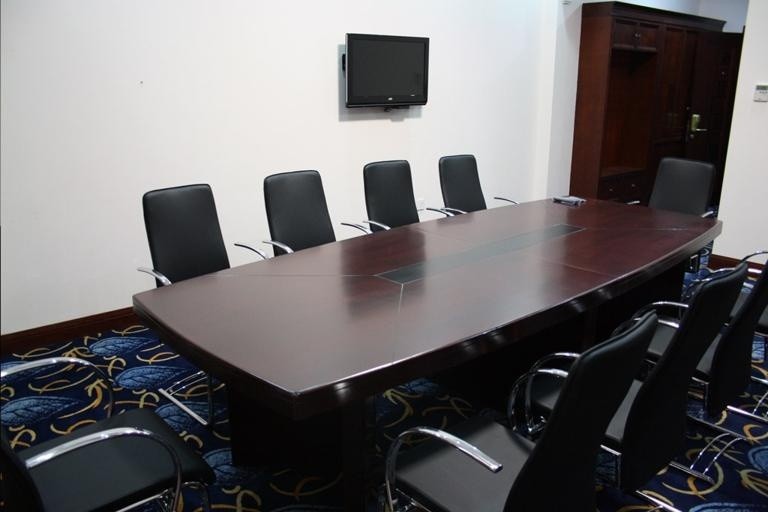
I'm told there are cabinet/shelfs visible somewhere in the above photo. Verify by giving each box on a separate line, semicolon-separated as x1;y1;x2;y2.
567;0;728;207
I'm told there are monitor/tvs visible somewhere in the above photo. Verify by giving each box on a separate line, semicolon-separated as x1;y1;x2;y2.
345;33;429;108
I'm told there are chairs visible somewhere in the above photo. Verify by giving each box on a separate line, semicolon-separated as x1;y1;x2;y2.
1;356;221;512
